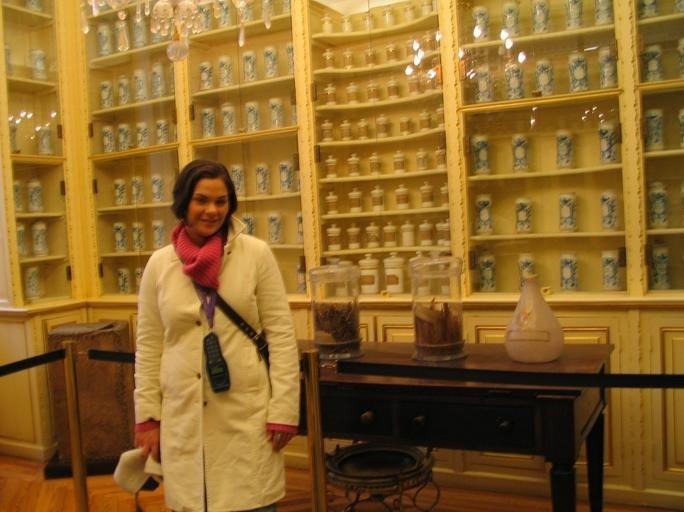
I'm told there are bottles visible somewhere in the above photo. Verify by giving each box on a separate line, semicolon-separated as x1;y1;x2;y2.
505;270;563;363
316;1;452;294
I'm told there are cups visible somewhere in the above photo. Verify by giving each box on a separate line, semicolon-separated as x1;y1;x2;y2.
97;11;178;296
635;0;682;292
194;0;306;295
469;0;621;290
3;1;54;301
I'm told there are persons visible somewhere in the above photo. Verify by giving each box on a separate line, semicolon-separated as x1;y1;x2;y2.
132;157;301;512
